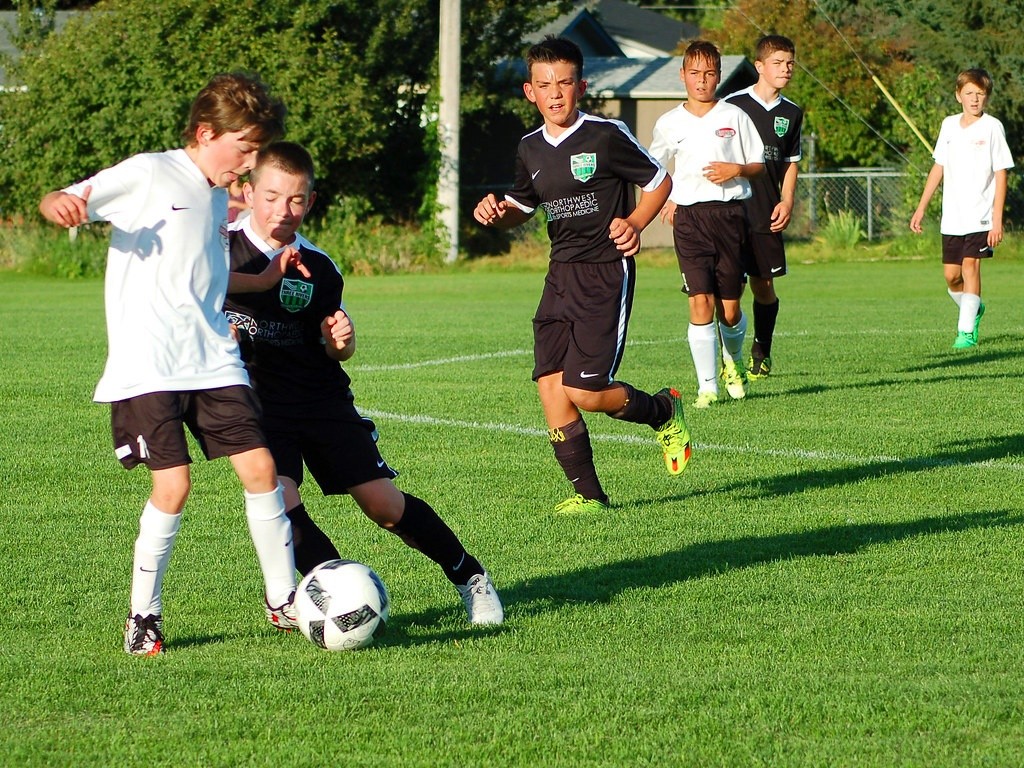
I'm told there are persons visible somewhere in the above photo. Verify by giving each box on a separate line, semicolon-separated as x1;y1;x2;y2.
659;35;804;380
649;41;766;407
473;41;693;514
39;73;311;654
224;142;506;626
910;68;1014;348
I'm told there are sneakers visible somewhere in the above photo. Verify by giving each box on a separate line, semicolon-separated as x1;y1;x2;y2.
555;494;609;514
265;591;303;634
973;303;985;342
693;393;717;409
651;388;692;475
952;331;977;348
454;571;504;625
124;611;166;658
748;355;770;381
720;346;748;399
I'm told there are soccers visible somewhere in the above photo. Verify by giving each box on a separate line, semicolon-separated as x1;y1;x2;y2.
293;559;389;650
